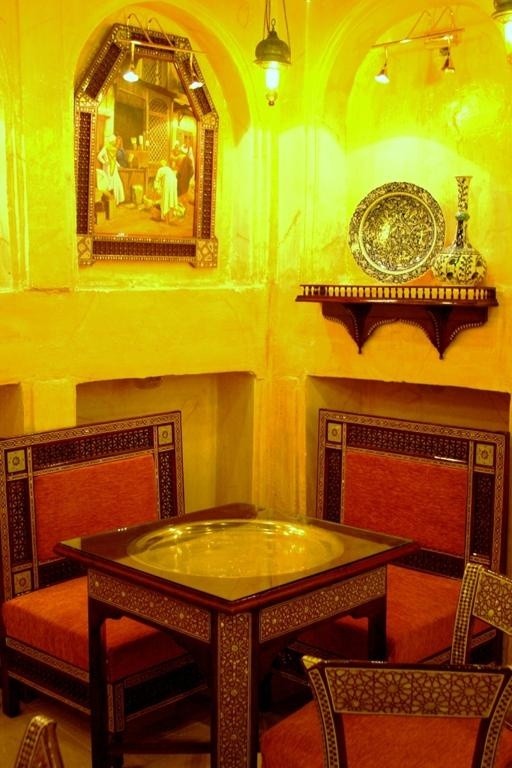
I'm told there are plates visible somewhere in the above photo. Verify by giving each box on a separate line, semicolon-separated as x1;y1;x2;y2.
347;182;446;286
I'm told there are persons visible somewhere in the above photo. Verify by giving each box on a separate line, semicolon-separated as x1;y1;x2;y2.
95;133;193;226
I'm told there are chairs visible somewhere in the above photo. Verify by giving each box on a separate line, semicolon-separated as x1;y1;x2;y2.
263;408;511;713
14;715;65;765
260;562;512;768
0;411;209;768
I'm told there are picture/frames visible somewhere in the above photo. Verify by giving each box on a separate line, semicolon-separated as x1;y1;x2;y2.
74;24;218;267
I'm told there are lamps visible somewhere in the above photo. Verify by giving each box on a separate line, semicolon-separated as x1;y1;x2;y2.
110;40;208;89
370;29;468;84
489;0;512;58
254;0;292;109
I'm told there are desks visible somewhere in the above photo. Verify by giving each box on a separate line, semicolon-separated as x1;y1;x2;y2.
56;503;421;768
